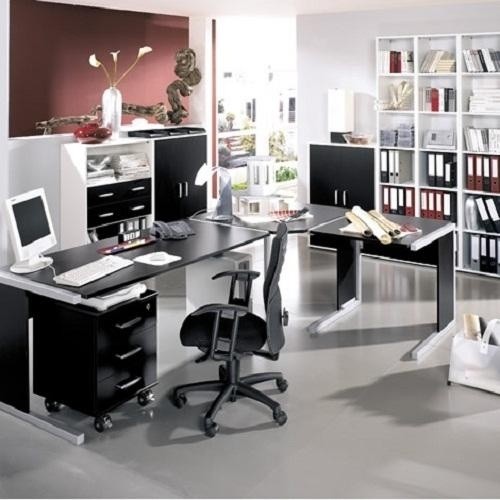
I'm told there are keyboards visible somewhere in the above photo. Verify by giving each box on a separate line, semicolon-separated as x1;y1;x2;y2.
52;254;134;287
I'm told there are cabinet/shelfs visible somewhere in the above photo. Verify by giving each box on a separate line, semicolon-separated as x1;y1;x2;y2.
459;31;500;278
308;143;374;257
153;133;207;223
374;36;417;264
418;32;459;268
60;137;154;252
32;289;162;433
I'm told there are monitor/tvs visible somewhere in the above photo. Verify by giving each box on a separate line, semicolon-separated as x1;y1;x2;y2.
4;187;57;274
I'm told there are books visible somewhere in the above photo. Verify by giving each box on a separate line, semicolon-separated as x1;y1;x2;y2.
86;151;151;188
378;46;500;275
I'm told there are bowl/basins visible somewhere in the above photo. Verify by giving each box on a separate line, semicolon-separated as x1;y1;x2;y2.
342;134;374;145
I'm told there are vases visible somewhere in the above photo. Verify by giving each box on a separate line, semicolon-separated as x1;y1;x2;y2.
102;86;123;132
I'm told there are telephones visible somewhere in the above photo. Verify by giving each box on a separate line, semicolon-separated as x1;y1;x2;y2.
153;221;196;240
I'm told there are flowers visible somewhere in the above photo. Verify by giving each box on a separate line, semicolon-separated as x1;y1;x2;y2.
89;45;153;128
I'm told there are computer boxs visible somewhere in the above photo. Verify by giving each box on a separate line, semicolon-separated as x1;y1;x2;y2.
186;250;252;317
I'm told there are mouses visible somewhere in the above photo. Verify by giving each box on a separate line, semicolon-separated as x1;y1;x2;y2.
147;251;169;261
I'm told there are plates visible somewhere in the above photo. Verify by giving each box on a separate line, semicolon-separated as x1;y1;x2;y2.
76;137;105;145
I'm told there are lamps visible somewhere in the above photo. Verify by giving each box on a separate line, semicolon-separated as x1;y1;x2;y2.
327;86;355;143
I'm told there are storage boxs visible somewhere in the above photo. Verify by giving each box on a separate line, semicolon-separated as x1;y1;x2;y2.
446;318;500;396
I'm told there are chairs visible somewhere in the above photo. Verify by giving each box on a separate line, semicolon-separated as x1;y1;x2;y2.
168;221;291;439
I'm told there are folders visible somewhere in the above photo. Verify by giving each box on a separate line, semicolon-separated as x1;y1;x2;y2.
382;186;414;218
475;197;500;234
380;150;412;185
470;234;500;275
466;155;500;193
420;189;457;227
427;153;457;188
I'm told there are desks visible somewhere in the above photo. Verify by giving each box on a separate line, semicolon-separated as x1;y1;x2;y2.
208;199;455;362
0;218;269;446
